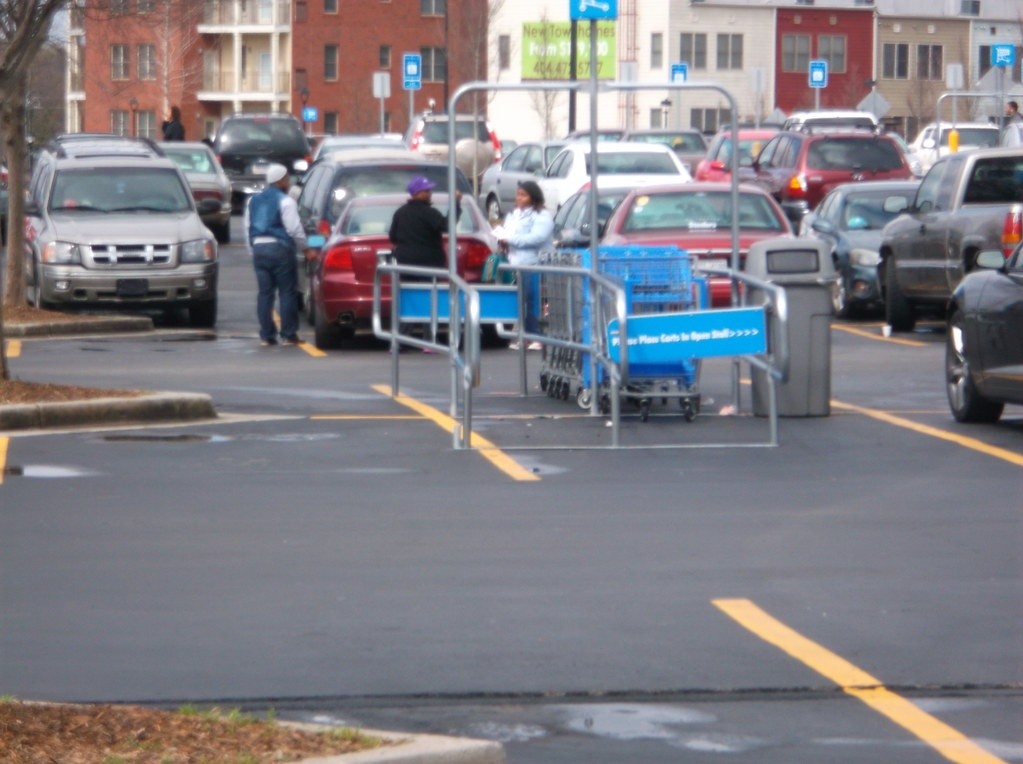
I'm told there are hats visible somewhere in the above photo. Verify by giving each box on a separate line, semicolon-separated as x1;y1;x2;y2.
266;163;287;184
406;177;437;195
517;180;545;206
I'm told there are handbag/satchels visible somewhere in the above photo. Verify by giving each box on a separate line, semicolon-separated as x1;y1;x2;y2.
481;240;508;282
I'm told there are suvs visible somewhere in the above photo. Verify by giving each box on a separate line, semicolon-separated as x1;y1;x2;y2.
198;110;311;215
0;130;233;329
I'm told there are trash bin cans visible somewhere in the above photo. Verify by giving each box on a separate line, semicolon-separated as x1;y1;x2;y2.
744;237;835;419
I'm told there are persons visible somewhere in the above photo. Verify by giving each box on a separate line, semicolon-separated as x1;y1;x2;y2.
1006;102;1023;123
496;180;555;350
161;106;185;142
245;163;319;346
388;175;463;354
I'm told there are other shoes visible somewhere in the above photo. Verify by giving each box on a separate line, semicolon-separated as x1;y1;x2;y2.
281;332;305;346
529;342;543;350
261;339;277;346
509;341;520;350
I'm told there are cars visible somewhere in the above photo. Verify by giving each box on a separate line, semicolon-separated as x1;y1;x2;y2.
286;111;1023;422
